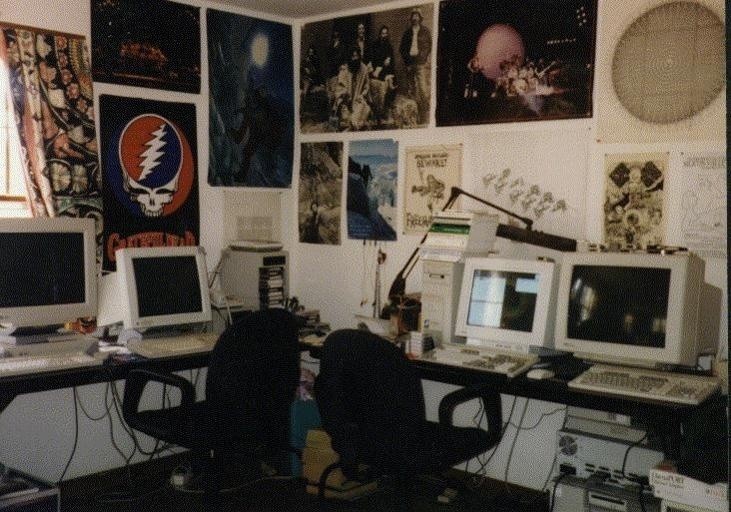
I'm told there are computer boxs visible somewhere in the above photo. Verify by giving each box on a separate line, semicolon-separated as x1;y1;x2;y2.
420;259;465;345
219;250;290;314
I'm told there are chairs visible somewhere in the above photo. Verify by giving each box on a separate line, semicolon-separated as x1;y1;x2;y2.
314;328;503;511
122;307;300;512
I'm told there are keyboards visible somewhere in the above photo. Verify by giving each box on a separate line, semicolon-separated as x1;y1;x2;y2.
420;342;539;379
126;332;218;360
567;363;721;406
0;344;105;378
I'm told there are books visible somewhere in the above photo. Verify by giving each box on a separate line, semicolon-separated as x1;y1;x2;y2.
648;458;730;512
417;208;500;263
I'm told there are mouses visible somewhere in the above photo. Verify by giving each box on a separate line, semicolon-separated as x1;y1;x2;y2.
527;368;554;380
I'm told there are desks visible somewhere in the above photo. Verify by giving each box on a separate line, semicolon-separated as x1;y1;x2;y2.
310;324;731;511
0;318;329;512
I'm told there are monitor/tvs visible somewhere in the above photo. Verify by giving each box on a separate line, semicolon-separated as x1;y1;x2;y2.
0;217;98;345
454;257;560;357
553;252;722;372
96;246;212;344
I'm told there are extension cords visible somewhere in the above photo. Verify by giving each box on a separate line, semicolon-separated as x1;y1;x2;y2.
170;462;194;487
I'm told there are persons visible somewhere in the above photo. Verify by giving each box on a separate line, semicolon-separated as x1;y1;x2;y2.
300;8;432;129
311;202;337;244
462;54;549;98
